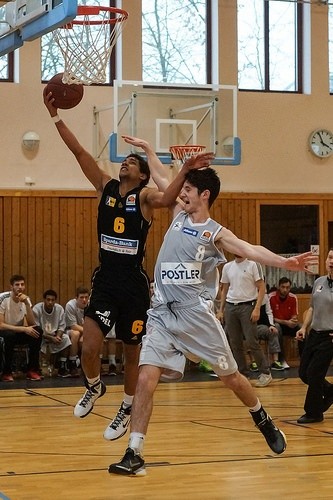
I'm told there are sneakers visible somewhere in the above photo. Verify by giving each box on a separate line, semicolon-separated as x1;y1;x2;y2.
271;360;284;371
108;447;146;477
26;370;41;381
281;360;290;368
254;373;272;387
69;366;81;377
121;365;124;374
1;373;14;382
100;366;108;376
249;361;259;372
254;413;287;455
37;368;45;379
199;359;213;372
73;380;107;419
108;363;117;376
103;400;132;441
58;367;71;378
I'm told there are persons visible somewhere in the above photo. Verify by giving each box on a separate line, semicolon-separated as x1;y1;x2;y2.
0;249;333;423
108;135;319;478
43;86;217;439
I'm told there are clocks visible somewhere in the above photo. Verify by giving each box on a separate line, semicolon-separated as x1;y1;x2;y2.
308;128;333;159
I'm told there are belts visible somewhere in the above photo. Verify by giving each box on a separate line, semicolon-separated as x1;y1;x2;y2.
225;300;255;305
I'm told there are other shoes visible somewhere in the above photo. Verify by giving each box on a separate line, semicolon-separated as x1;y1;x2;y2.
297;412;323;423
322;383;333;413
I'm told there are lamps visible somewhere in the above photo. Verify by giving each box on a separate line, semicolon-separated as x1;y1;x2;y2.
21;130;41;152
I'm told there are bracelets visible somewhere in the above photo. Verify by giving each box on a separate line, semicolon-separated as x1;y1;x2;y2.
51;114;62;123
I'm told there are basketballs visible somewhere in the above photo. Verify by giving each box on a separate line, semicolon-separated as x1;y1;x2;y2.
46;72;84;110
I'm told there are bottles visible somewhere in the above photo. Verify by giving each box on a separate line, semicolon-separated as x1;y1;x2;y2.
48;365;52;377
